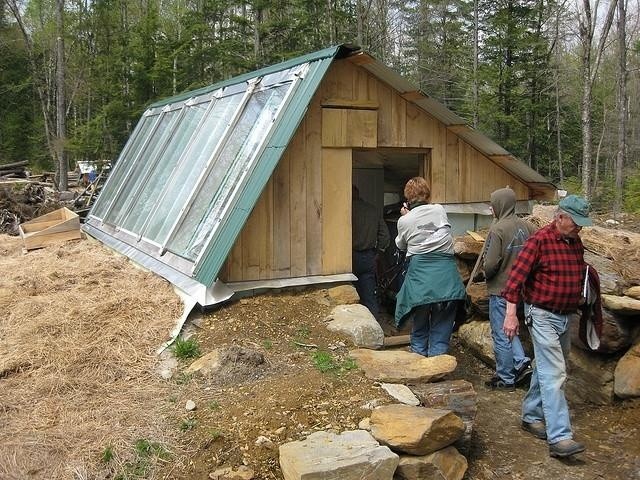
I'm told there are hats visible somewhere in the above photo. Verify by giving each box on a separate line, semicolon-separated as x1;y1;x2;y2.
559;195;592;227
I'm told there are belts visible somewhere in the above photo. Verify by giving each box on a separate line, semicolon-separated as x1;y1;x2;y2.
534;305;569;315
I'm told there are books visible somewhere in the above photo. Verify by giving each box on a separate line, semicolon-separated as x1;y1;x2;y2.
583;263;590;306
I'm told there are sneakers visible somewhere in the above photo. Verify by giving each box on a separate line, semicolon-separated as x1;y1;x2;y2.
549;439;584;457
485;361;533;390
522;421;547;439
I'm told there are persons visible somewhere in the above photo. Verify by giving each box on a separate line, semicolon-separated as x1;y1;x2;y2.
395;175;467;357
500;194;592;457
351;184;391;321
469;187;536;391
